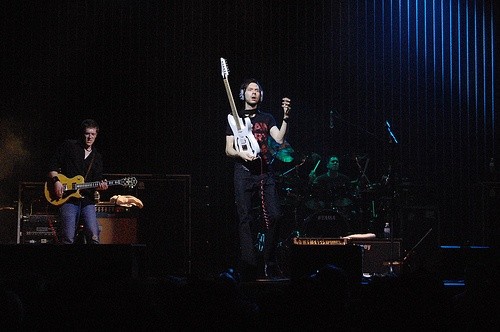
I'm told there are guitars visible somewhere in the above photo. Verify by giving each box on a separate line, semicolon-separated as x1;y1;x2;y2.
220;57;262;162
44;173;138;206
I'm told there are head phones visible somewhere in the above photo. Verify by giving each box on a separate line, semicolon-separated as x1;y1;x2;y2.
238;79;263;102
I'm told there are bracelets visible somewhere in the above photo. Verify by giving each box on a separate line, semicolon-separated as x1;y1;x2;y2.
283;118;289;123
51;176;59;185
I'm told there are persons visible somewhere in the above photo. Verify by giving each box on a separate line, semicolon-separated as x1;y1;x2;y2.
308;155;350;190
0;271;500;332
47;119;108;243
225;80;291;282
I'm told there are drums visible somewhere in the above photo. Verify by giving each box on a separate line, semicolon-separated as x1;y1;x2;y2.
305;182;325;210
277;168;303;206
375;175;400;200
324;182;354;208
298;209;349;243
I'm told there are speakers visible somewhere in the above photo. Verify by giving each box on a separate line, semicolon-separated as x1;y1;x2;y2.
435;245;492;282
83;202;138;244
350;239;401;275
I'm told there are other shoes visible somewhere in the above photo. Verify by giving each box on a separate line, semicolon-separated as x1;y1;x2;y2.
266;264;285;278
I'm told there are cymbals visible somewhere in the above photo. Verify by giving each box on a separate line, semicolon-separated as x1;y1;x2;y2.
341;152;367;160
290;149;313;162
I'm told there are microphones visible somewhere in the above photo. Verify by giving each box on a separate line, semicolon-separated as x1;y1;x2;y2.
384;121;398;144
